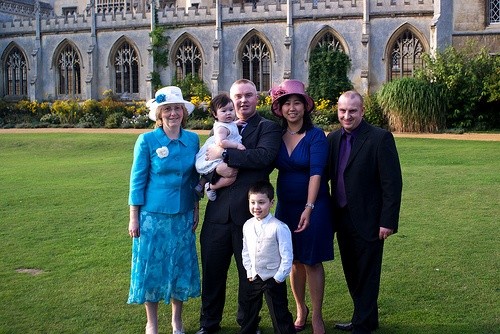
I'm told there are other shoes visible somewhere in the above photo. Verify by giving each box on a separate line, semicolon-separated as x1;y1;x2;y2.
204;181;217;201
193;186;204;198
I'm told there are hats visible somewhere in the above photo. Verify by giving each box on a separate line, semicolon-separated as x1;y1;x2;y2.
145;86;196;122
268;79;315;118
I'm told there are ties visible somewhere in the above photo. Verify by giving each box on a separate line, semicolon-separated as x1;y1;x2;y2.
337;133;353;207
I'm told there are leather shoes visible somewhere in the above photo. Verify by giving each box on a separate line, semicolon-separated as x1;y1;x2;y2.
333;322;354;331
194;325;221;334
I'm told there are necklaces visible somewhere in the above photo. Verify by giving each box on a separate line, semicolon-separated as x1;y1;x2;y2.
287;128;297;135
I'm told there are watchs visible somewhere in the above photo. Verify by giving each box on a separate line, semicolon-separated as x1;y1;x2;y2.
222;149;227;159
304;203;315;209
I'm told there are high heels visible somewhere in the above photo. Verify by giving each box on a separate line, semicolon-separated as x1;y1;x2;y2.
311;319;326;334
294;305;309;332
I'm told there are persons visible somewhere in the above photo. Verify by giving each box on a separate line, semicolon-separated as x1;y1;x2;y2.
195;93;246;200
236;181;297;334
194;78;282;334
326;90;402;334
269;80;334;334
126;85;204;334
